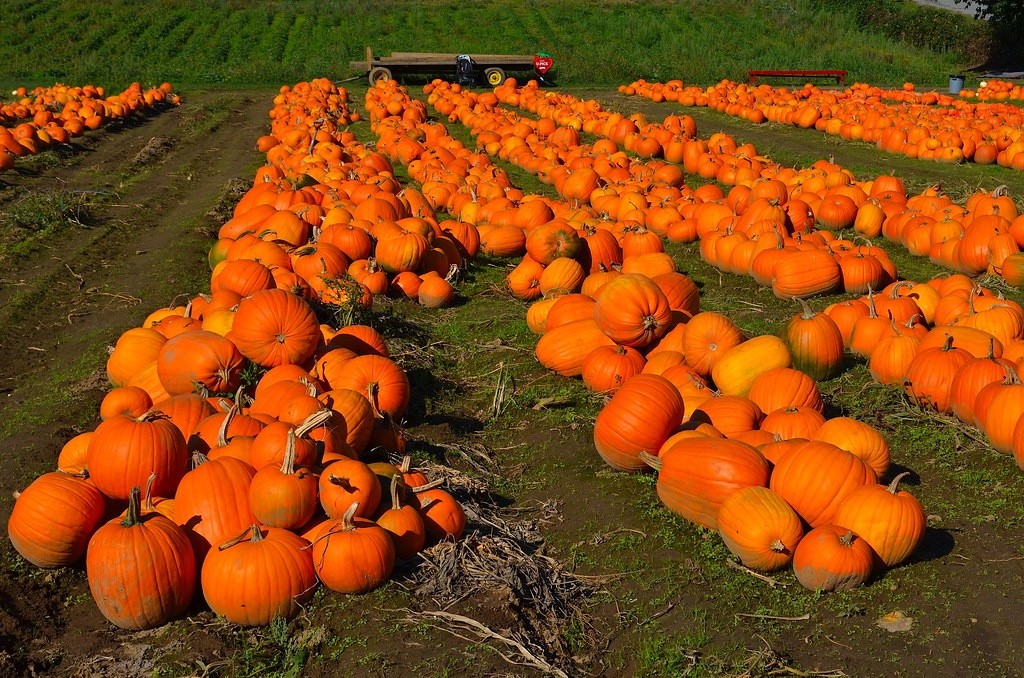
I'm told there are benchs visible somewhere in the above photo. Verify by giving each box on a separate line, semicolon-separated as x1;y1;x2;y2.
749;70;845;86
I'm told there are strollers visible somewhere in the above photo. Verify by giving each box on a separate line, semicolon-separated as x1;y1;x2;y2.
455;55;477;89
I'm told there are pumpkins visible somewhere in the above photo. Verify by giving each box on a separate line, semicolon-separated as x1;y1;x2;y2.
0;78;1024;631
